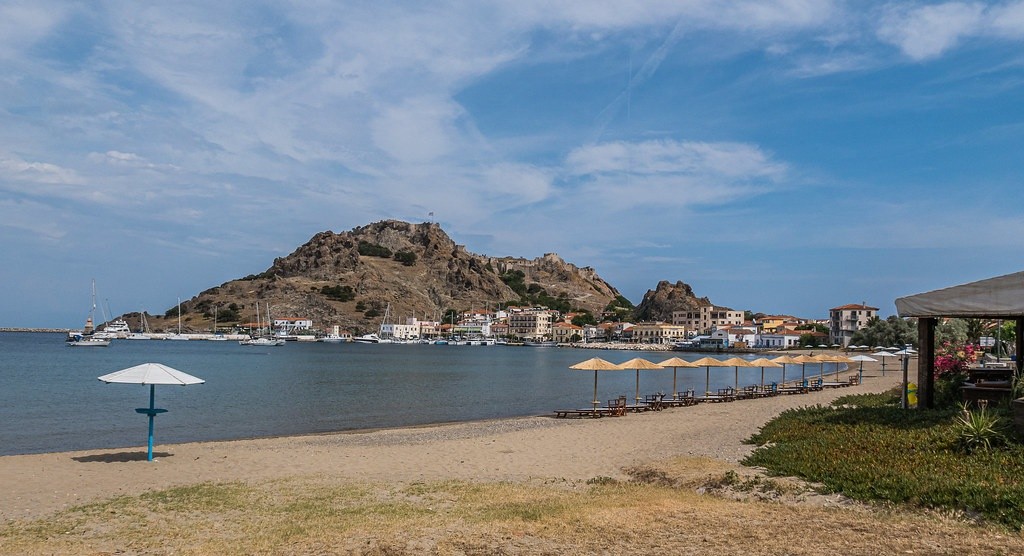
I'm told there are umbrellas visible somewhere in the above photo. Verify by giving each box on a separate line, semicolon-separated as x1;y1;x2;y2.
874;346;885;352
804;344;813;350
617;357;665;405
723;355;757;398
815;354;839;392
98;363;206;462
886;346;900;353
850;354;879;384
833;356;857;389
901;349;918;353
893;351;912;369
848;345;858;351
656;356;701;400
859;345;869;352
751;357;784;397
870;350;899;376
818;344;828;351
569;356;626;412
794;353;823;392
831;344;841;351
690;356;731;403
770;354;803;394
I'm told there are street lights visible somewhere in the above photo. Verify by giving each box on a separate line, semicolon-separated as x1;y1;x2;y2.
894;338;908;410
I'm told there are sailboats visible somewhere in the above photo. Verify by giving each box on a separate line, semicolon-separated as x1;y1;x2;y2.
64;279;557;347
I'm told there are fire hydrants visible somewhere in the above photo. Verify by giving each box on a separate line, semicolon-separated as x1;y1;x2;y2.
907;383;917;406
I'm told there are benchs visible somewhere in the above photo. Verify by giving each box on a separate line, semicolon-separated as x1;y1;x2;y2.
608;374;860;417
554;409;602;419
